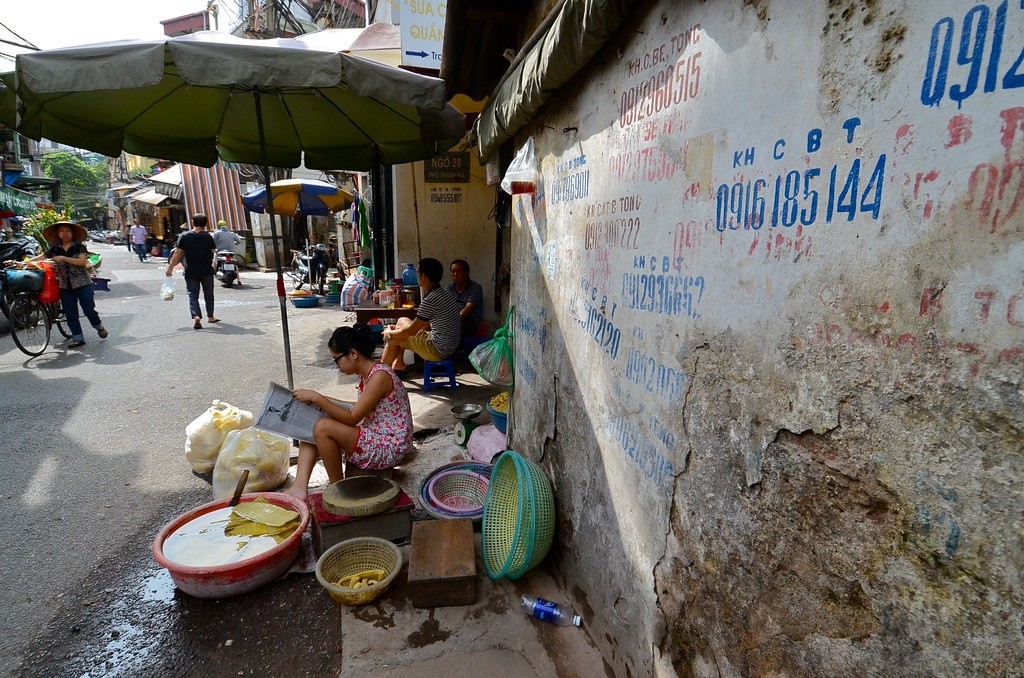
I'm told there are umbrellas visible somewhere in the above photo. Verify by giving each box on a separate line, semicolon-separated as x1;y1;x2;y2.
0;29;466;390
238;177;356;222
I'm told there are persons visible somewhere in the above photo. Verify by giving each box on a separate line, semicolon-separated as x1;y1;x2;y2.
165;213;220;330
211;220;242;285
128;217;148;262
380;258;461;377
269;407;286;418
278;322;413;502
445;259;484;345
3;221;108;347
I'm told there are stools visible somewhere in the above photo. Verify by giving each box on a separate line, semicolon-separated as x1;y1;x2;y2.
303;487;416;559
474;321;490;337
344;458;393;479
423;357;456;393
408;517;477;609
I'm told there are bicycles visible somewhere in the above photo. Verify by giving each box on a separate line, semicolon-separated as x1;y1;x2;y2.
2;259;74;356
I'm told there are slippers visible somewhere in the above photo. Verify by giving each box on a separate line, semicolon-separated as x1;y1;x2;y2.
194;319;203;330
208;318;219;323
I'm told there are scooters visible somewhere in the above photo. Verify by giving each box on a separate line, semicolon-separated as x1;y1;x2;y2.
212;243;239;288
287;243;335;295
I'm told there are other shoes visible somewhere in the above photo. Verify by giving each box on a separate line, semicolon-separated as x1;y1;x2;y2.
97;326;108;338
68;341;85;348
238;281;242;285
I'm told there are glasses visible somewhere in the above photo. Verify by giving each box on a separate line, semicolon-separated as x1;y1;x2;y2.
333;352;348;367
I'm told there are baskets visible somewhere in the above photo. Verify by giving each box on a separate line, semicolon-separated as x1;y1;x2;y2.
417;453;556;581
315;536;403;605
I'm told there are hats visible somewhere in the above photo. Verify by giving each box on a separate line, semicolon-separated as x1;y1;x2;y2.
43;221;87;244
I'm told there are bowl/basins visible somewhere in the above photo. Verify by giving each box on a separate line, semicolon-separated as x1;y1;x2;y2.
287;292;320;307
153;492;310;596
485;399;507;434
326;292;340;303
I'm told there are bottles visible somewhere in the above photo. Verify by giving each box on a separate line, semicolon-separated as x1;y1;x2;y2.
519;593;582;627
378;289;395;306
401;286;421;307
403;263;418;286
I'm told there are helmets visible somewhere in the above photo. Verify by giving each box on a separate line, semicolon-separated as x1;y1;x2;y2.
217;220;228;227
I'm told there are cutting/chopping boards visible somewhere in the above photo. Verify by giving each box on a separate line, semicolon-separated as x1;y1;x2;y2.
322;475;400;516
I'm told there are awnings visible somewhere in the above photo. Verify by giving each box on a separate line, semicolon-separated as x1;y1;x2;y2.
106;183;139;207
128;187;170;217
147;162;184;201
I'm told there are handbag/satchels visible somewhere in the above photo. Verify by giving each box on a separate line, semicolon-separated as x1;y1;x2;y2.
161;276;175;301
40;266;61;304
468;305;515;389
184;399;255;474
211;425;290;501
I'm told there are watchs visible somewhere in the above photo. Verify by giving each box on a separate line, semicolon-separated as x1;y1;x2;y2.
387;331;392;339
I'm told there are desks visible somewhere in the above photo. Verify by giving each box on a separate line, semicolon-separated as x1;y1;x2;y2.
354;298;419;374
